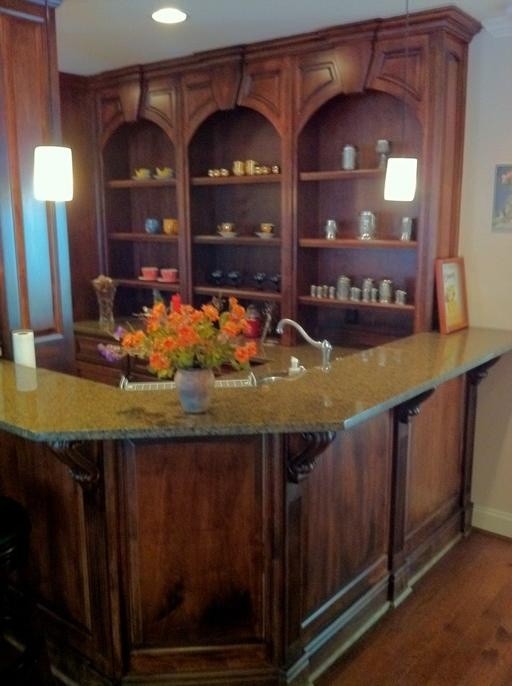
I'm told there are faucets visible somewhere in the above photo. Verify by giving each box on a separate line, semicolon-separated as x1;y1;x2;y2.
276;318;332;365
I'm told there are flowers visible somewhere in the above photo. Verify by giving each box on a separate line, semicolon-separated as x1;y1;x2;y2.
95;295;262;379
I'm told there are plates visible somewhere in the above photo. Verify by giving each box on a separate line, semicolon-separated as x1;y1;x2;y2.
217;232;239;237
256;232;276;239
130;175;150;180
137;277;156;281
153;175;173;179
156;278;176;283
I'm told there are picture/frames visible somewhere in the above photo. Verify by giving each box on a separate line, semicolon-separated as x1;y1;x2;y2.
432;251;470;336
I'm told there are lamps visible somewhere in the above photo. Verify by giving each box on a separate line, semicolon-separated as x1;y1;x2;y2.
28;139;76;206
379;1;421;205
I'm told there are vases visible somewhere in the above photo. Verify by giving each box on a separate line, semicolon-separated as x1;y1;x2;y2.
170;364;217;416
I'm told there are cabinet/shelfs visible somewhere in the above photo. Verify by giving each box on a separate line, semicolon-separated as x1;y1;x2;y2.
185;172;283;344
74;319;263;387
103;176;181;321
296;166;418;349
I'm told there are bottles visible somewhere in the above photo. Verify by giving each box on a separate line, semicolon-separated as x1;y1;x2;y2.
163;218;179;235
311;274;408;304
401;217;412;241
144;218;161;234
243;304;260;337
340;145;355;170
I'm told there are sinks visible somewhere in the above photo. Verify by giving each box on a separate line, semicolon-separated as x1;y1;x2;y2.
256;372;288;384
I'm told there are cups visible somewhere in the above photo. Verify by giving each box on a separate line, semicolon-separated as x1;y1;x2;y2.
218;222;236;232
141;266;158;279
135;166;149;176
159;269;177;281
326;220;336;239
260;223;279;233
208;159;279;176
157;167;173;175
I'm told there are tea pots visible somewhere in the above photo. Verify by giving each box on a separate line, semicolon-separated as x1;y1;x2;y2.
356;211;376;238
